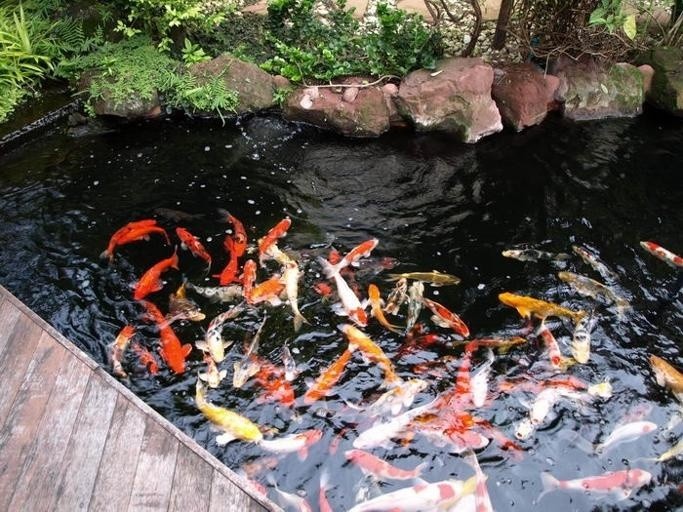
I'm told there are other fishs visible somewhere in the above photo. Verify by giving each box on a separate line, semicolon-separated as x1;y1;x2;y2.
99;208;683;512
